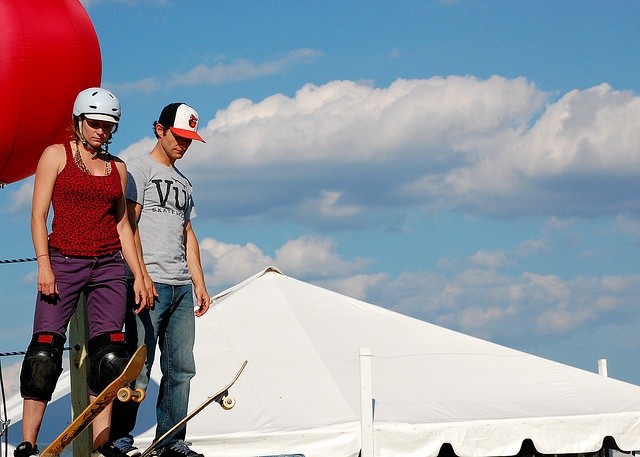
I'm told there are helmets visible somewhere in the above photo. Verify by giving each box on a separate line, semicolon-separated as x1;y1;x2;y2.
72;87;122;124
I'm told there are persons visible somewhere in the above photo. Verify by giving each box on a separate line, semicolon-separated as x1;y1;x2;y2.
14;87;148;457
109;102;210;457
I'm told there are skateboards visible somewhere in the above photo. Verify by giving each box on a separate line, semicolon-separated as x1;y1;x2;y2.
142;360;248;456
40;344;148;456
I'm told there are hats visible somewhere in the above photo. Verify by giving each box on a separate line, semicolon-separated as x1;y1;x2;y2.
158;103;207;144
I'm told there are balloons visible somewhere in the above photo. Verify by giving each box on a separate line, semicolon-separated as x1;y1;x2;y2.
0;0;102;189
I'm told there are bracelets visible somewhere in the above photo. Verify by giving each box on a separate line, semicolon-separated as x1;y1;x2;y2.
37;253;50;258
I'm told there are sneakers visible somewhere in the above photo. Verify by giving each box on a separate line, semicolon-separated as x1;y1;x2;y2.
114;439;142;457
15;442;39;457
154;440;204;457
90;442;128;457
